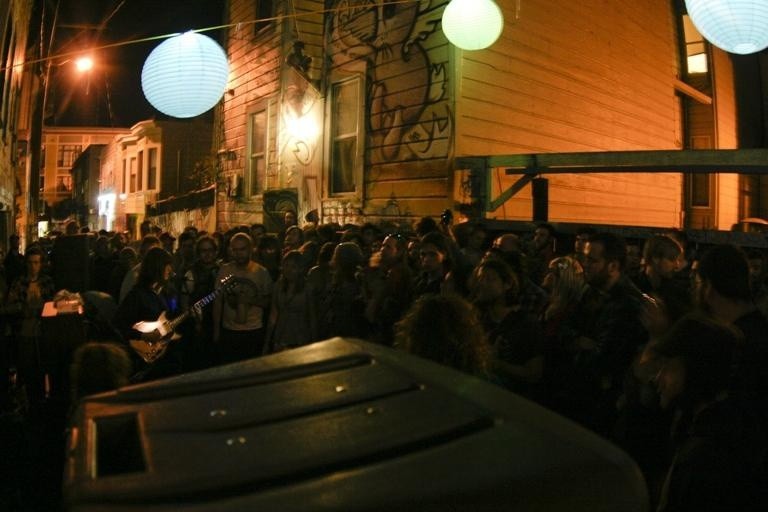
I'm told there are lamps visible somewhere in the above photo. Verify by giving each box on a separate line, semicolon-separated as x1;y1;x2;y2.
441;0;505;50
140;29;229;118
683;0;768;56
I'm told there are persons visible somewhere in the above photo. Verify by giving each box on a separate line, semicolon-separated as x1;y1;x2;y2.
69;338;130;407
383;218;768;511
2;210;382;392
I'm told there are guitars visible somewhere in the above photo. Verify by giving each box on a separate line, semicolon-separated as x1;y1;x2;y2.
130;273;240;364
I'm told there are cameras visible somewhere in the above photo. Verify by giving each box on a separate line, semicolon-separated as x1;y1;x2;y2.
441;209;451;225
